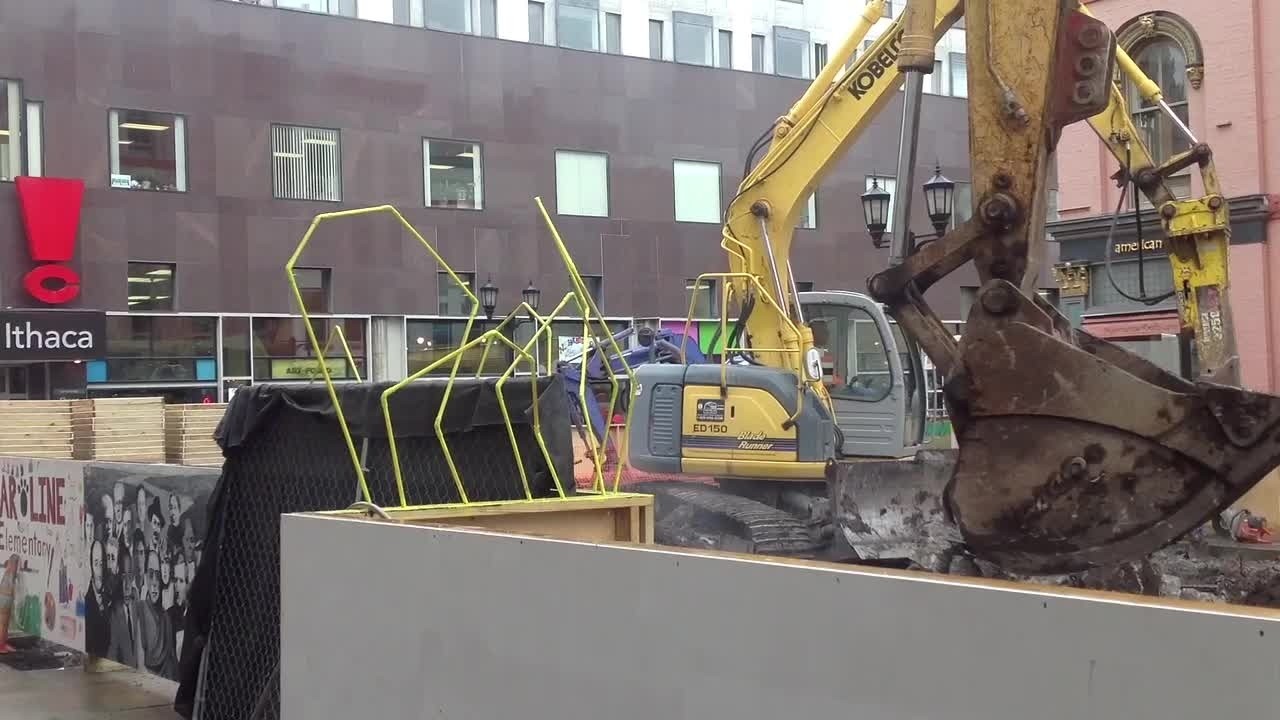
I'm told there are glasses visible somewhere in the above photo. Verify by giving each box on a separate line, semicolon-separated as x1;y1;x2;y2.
146;568;160;578
121;572;133;581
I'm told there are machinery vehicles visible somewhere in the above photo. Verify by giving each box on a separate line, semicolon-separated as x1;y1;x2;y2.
578;1;1279;599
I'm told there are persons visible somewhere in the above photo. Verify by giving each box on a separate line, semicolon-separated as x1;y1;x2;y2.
85;483;205;672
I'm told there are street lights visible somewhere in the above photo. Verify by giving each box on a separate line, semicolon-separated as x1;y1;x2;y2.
859;161;956;412
